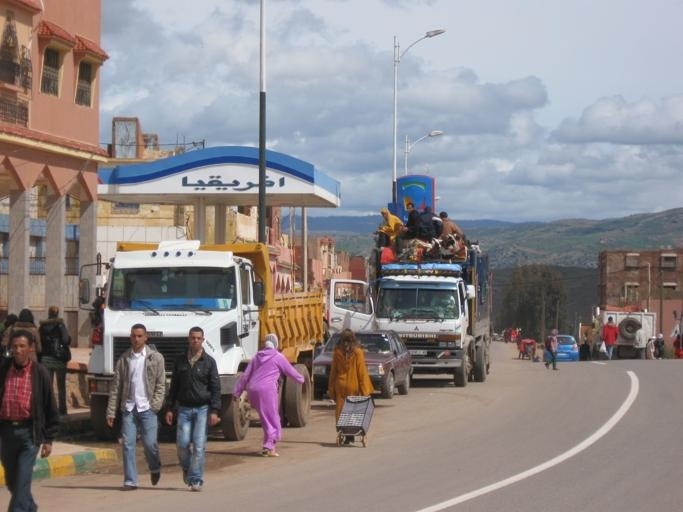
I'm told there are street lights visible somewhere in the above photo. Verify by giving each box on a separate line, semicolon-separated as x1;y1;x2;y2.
390;27;445;207
402;130;444;176
642;260;650;311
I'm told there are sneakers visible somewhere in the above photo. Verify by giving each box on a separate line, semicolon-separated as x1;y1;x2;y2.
120;470;201;491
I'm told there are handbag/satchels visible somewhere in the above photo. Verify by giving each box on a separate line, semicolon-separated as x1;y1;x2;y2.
51;337;71;362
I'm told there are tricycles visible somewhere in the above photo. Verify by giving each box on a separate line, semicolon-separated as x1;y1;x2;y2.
519;339;536;360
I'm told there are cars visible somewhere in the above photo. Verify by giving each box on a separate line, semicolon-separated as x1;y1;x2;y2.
542;334;579;361
311;329;415;401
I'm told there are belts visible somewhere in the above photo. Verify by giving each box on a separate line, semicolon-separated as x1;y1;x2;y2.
3;420;24;427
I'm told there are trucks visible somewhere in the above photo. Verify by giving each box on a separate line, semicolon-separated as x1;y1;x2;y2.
328;248;491;387
590;304;657;359
76;239;325;440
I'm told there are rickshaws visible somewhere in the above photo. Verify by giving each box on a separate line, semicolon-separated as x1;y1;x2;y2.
335;394;375;448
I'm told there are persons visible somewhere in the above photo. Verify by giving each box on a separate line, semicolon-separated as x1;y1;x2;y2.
502;314;681;370
1;308;43;355
1;331;55;510
231;335;305;458
104;322;169;491
327;327;373;447
435;290;456;306
80;283;106;373
164;329;221;491
375;202;483;266
3;312;18;327
36;306;70;414
